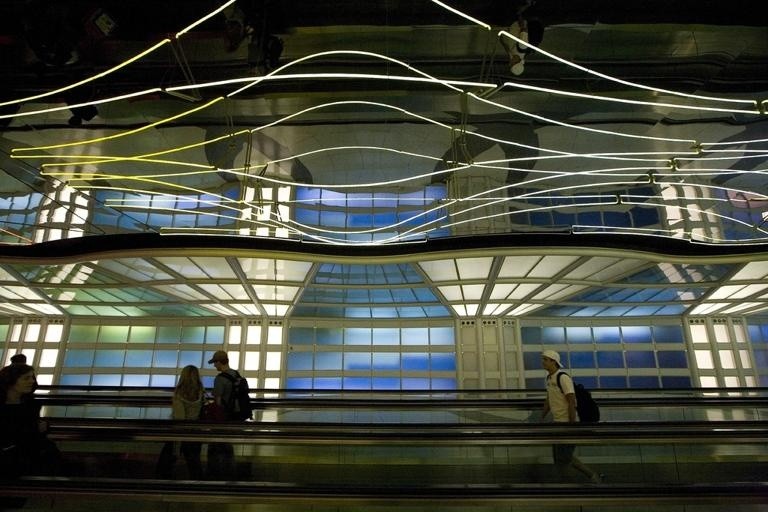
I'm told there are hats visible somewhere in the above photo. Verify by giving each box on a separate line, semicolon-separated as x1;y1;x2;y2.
206;351;229;365
540;349;564;369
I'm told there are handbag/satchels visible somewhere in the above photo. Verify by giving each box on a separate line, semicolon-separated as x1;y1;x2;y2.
200;402;220;424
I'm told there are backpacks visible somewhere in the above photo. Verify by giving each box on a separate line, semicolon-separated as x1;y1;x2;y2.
556;371;600;424
218;369;253;421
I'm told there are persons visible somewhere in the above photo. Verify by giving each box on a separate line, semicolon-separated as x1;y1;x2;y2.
1;362;57;480
10;354;39;394
153;365;207;480
538;349;599;483
202;350;243;481
